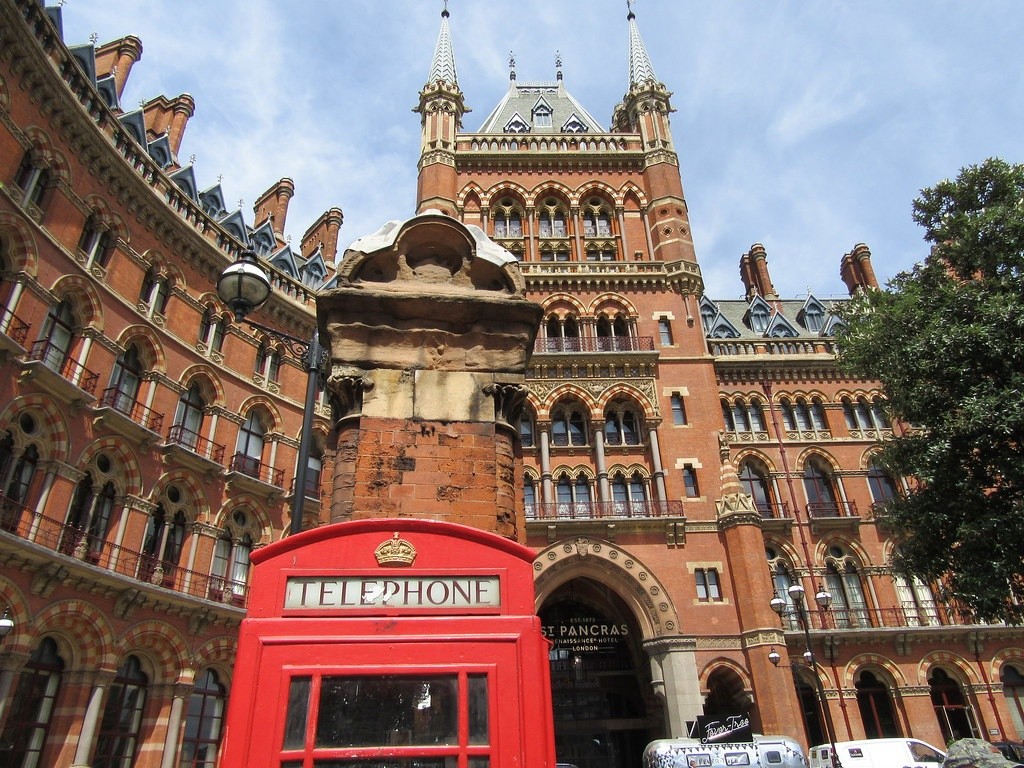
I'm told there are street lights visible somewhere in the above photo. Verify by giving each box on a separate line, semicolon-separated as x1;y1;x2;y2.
212;243;322;538
767;644;816;748
771;575;842;768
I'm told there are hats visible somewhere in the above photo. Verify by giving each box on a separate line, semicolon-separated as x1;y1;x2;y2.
942;738;1024;768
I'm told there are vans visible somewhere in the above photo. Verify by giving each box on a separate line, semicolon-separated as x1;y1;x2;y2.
808;737;948;768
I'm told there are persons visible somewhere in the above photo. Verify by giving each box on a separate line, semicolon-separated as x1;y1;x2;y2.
942;738;1024;768
